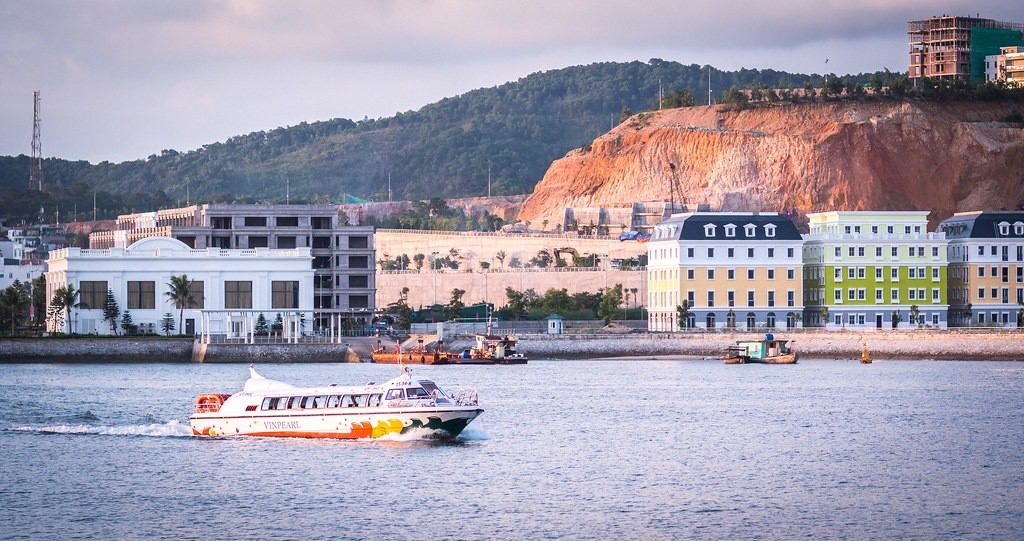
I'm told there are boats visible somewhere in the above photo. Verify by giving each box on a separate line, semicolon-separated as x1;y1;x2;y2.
446;305;529;365
723;344;751;365
372;338;459;366
190;364;485;444
859;343;873;364
736;338;800;364
